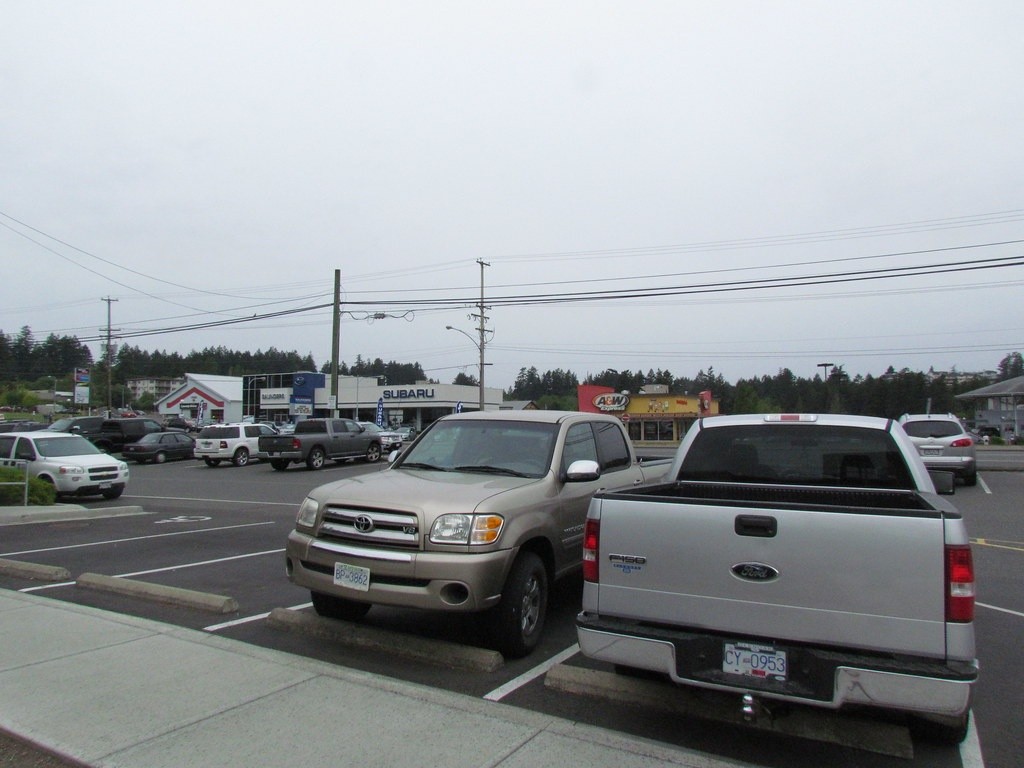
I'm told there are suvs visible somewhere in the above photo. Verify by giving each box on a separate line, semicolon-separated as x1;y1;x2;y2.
0;430;130;501
346;421;403;452
891;413;977;489
193;421;282;467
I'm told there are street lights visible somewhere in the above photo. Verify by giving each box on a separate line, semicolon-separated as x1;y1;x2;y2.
47;375;56;416
817;362;836;385
247;376;266;416
445;325;485;411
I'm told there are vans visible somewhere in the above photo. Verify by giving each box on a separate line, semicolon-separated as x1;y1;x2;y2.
35;415;107;448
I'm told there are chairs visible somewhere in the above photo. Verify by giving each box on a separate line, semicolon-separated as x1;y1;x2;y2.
696;446;777;505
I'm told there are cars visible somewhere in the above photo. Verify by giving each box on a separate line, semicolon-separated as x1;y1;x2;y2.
120;409;148;419
122;429;197;465
258;419;297;435
963;422;1000;444
161;416;239;434
395;426;417;441
0;418;53;433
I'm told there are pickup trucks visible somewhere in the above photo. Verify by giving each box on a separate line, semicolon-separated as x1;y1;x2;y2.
69;416;188;456
284;409;677;661
571;414;981;748
256;417;383;471
